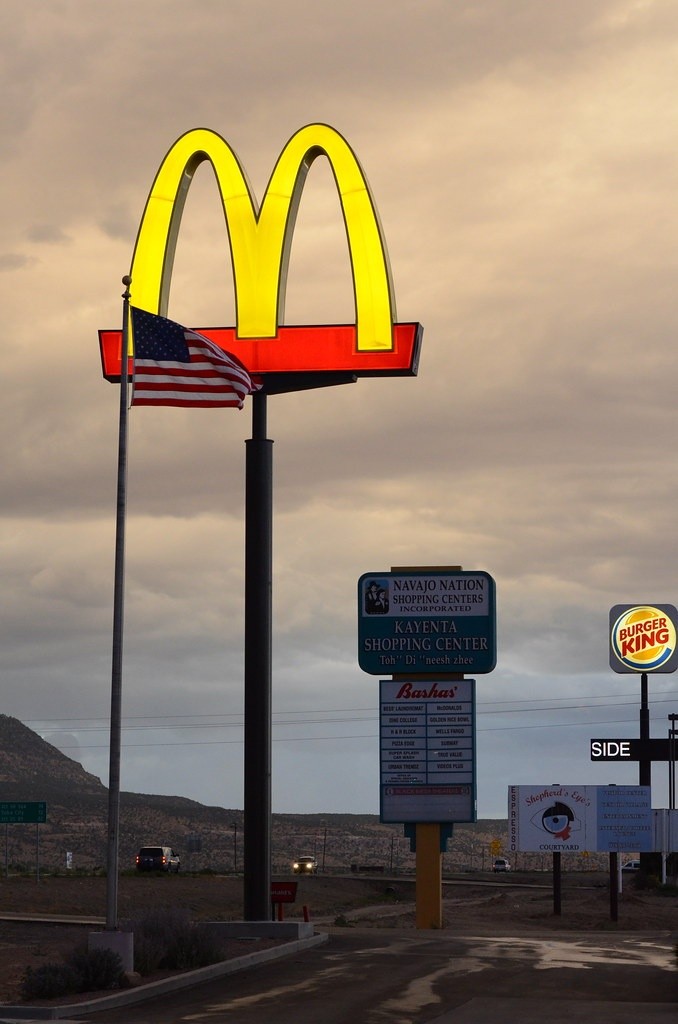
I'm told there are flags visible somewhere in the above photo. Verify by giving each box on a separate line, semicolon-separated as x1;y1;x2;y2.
127;305;263;413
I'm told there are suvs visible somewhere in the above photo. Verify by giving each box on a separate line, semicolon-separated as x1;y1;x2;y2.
493;860;511;873
136;846;181;875
294;856;318;875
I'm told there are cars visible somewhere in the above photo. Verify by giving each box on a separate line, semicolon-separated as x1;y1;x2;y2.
621;860;640;874
